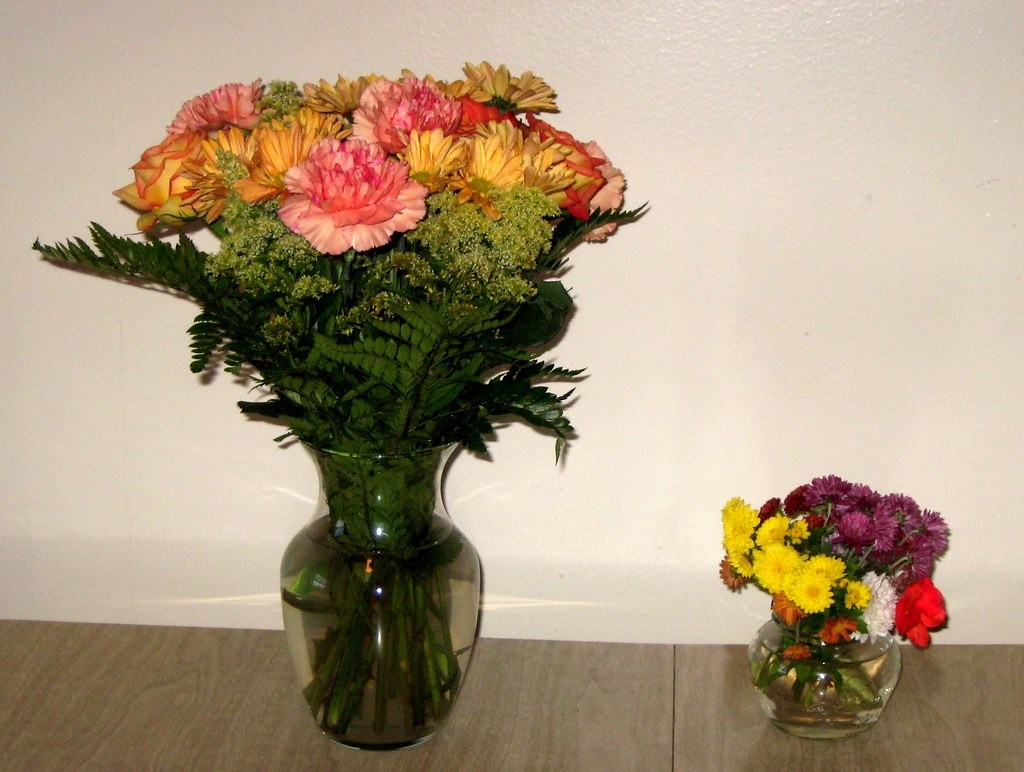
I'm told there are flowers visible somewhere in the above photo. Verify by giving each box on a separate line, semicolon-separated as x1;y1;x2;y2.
31;61;651;737
720;474;950;709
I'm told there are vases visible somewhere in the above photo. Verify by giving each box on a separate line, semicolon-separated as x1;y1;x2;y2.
279;436;483;751
747;611;901;742
